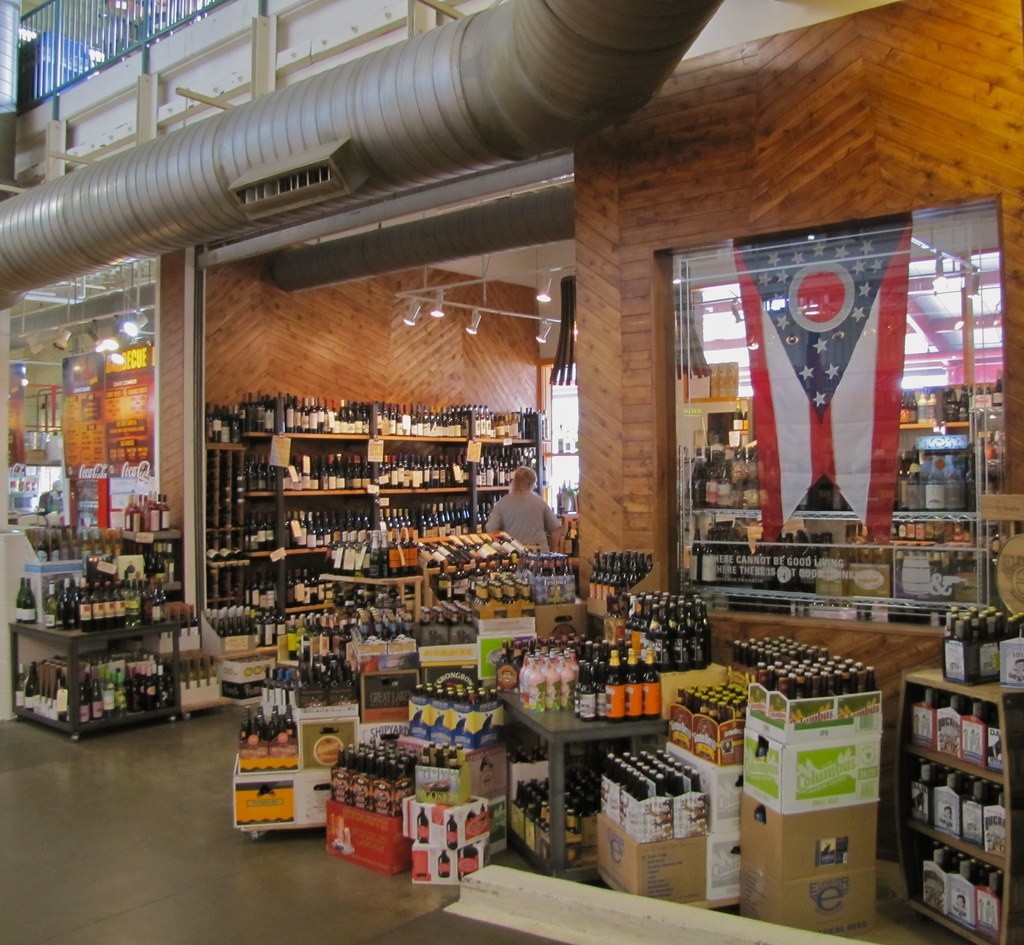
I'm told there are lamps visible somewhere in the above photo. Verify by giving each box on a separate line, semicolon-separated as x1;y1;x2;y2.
25;308;148;354
394;254;745;343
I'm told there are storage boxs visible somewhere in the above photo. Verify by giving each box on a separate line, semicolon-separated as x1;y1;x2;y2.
25;528;882;937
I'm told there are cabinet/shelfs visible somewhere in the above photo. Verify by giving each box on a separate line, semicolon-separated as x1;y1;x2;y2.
243;392;543;620
676;444;979;612
893;668;1024;945
205;442;249;609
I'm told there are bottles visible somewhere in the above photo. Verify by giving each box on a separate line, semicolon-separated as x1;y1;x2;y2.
9;389;1024;945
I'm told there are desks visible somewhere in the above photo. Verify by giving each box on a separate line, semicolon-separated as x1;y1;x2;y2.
8;621;181;742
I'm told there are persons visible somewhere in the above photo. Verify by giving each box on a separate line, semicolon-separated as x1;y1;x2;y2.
39;480;63;514
485;465;563;553
940;806;952;827
1008;659;1024;682
953;895;967;918
